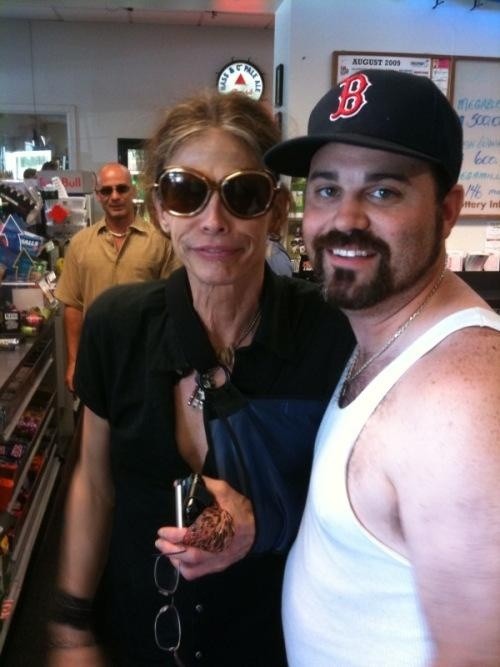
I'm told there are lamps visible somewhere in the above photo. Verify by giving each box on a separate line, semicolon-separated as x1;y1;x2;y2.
31;127;40;148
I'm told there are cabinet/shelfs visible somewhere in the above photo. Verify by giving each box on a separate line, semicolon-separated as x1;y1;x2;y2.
0;311;68;663
279;169;307;273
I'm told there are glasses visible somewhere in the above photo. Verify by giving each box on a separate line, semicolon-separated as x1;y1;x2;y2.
154;540;187;667
153;165;281;219
100;183;130;196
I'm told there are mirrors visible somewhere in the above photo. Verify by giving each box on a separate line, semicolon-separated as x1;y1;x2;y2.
0;106;79;184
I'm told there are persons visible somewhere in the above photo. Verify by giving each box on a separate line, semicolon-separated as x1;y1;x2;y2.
51;160;186;396
265;183;296;278
45;90;360;666
259;69;500;666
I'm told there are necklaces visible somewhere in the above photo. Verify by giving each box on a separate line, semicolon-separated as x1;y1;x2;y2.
340;265;447;405
188;303;260;411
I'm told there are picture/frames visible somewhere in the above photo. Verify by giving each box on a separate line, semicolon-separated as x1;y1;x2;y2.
275;62;285;107
274;111;283;133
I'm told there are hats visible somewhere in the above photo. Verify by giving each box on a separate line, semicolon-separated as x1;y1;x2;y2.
265;68;463;178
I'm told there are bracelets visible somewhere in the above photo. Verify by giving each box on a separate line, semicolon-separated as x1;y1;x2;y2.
46;637;96;651
47;591;91;631
